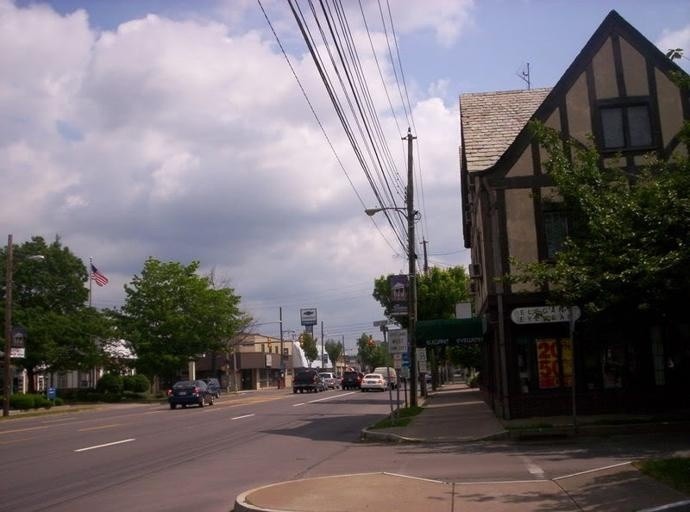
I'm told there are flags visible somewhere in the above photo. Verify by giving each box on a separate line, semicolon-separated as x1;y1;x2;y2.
89;263;109;289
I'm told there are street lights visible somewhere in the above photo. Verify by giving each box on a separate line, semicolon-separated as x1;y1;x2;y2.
231;319;284;393
364;206;420;407
3;234;45;418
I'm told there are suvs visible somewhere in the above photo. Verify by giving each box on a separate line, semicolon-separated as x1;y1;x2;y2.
199;377;220;399
341;371;364;390
293;368;322;394
319;371;340;390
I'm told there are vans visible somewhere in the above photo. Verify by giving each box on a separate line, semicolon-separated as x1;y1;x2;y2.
374;367;398;390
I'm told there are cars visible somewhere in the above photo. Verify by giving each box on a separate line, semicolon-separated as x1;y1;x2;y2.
361;373;388;393
167;379;215;409
